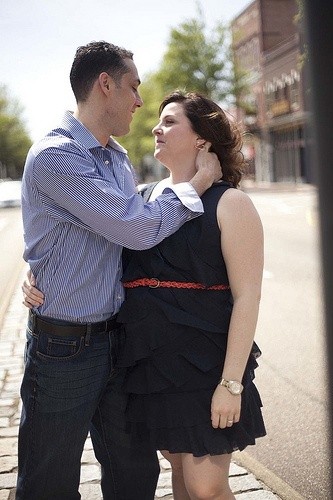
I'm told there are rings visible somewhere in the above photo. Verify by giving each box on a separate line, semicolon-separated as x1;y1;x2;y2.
228;421;234;423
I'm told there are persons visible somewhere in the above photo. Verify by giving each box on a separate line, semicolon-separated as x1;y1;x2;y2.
15;41;223;499
22;90;267;500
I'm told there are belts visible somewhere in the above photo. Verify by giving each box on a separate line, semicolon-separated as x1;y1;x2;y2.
27;308;122;338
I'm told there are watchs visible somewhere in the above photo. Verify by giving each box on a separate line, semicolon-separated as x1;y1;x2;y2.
217;377;243;394
